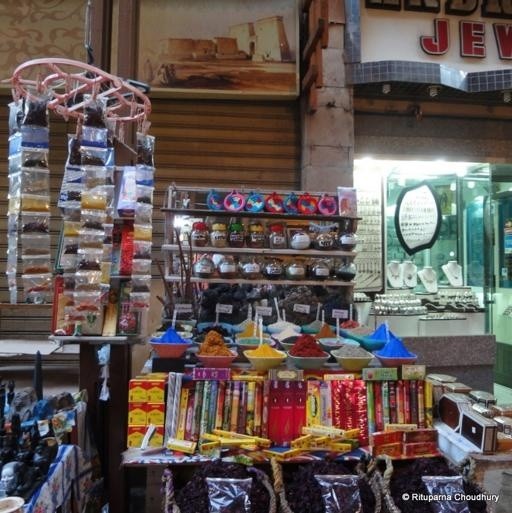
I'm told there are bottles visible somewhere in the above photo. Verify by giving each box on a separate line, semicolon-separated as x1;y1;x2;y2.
187;221;359;282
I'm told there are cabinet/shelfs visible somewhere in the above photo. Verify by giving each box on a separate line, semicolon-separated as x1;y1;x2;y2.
160;186;362;288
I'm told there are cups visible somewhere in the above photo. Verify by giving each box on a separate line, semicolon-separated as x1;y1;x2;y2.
168;37;240;58
1;494;26;513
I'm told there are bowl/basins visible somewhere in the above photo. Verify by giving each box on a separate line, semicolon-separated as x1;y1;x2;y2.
152;314;418;368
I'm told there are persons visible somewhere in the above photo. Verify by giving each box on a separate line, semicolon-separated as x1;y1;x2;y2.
403;260;417;288
441;260;463;286
417;265;438;293
387;260;403;288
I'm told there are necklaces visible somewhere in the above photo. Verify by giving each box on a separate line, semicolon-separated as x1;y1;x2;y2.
446;265;460;280
403;268;417;280
420;270;435;284
388;264;402;280
